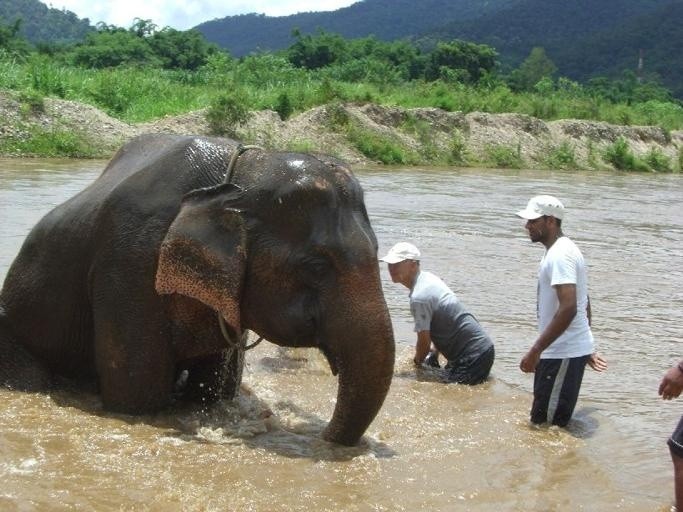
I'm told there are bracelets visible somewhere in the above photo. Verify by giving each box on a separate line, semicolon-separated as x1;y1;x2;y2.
677;362;683;372
413;358;422;365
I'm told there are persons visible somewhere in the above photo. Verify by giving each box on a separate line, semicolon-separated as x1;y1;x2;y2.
514;195;609;428
379;242;495;386
657;360;682;511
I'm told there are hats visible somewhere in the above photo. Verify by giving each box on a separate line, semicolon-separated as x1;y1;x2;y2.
376;241;422;268
514;195;564;223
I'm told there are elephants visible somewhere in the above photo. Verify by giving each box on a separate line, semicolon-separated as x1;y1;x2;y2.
0;132;397;447
